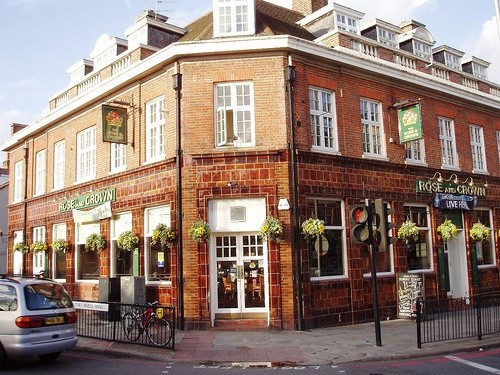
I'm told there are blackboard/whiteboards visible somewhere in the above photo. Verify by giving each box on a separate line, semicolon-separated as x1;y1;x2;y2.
395;272;426;319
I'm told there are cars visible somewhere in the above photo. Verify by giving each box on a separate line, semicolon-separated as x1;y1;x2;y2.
0;274;79;373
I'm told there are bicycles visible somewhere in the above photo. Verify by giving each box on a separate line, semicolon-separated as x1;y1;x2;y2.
122;300;173;347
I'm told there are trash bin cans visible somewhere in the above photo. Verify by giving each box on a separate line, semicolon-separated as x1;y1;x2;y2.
98;277;120;321
120;275;147;319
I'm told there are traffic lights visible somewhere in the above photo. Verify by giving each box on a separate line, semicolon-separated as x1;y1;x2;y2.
371;198;398;254
347;204;371;244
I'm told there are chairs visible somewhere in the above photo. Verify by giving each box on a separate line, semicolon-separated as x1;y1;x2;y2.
251;277;264;300
222;278;235;296
